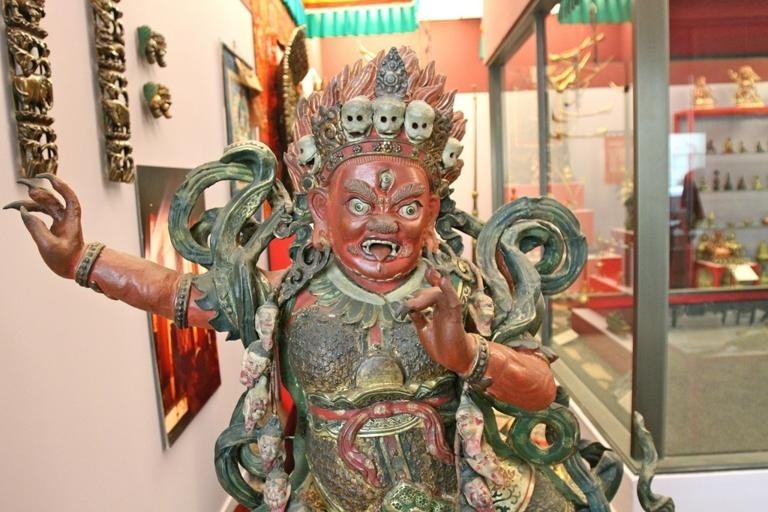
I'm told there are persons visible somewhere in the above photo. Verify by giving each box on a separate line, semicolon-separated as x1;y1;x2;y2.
2;43;591;512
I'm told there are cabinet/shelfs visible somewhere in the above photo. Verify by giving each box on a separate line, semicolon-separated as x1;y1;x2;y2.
673;106;768;262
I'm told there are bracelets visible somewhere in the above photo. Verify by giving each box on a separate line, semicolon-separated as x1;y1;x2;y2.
457;331;489;383
74;240;103;288
